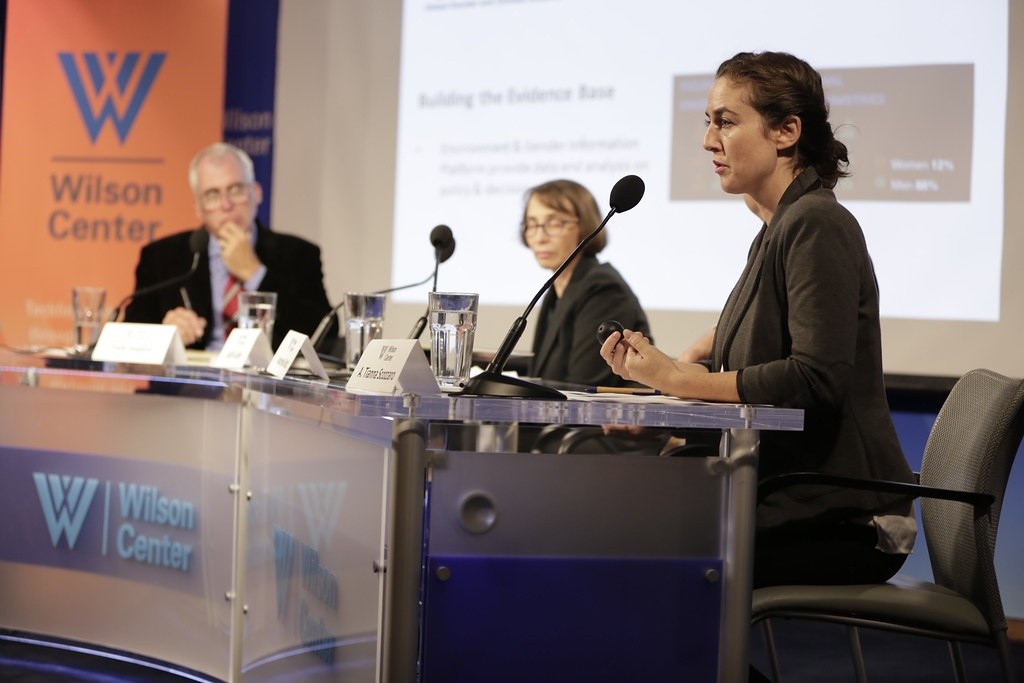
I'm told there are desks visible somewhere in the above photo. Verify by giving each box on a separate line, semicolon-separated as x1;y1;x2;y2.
0;349;804;683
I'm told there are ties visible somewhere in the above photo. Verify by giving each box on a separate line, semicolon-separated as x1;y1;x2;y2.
221;272;244;339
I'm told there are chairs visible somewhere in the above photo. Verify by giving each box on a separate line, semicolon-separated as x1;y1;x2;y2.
751;368;1023;683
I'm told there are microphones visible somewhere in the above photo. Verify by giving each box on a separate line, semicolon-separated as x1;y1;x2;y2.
407;225;453;340
73;228;209;359
461;175;645;399
285;238;456;374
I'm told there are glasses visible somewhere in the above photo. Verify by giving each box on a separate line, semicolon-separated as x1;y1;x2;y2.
195;184;251;209
520;218;580;236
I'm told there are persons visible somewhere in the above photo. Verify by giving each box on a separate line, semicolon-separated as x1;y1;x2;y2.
121;141;340;363
520;178;660;395
601;50;920;592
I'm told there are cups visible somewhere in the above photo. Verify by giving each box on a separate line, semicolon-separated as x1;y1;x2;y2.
235;290;277;350
70;287;106;354
428;290;480;394
342;292;387;379
